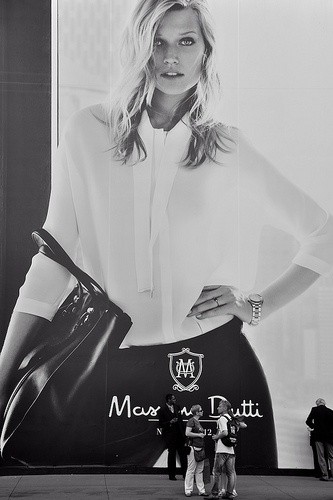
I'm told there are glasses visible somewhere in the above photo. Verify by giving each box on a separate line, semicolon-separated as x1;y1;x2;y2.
199;410;203;412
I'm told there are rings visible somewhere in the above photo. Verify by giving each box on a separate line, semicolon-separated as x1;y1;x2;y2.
213;298;220;308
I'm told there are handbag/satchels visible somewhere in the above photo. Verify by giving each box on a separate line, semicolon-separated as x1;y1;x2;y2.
193;447;207;462
1;226;135;473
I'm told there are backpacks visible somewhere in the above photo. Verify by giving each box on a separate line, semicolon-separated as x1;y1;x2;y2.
217;413;240;448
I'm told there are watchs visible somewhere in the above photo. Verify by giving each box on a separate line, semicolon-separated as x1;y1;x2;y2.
246;292;264;327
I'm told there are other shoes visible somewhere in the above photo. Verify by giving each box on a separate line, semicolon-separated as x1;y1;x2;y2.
169;476;177;481
199;491;209;497
186;491;192;496
211;487;238;498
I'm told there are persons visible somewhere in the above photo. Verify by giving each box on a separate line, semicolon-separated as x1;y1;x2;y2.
158;394;248;500
306;397;333;481
1;1;333;476
